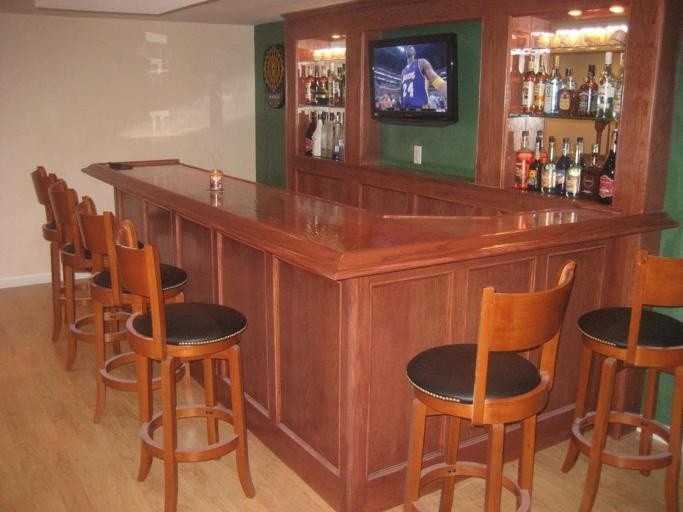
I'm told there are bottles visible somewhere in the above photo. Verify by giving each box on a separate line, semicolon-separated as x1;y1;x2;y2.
302;110;345;161
209;192;224;209
504;131;515;189
298;64;306;105
514;128;620;205
507;54;521;115
209;168;223;191
521;52;626;121
303;63;346;105
516;210;577;230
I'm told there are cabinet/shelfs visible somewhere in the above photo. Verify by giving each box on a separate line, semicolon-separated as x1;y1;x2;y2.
499;0;629;207
288;31;350;162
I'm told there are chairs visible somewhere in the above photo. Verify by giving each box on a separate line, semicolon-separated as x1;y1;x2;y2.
30;164;101;345
108;216;257;512
559;244;683;512
69;191;191;429
43;174;144;372
389;258;579;511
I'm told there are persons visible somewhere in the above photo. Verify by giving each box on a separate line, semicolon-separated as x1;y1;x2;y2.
375;43;448;112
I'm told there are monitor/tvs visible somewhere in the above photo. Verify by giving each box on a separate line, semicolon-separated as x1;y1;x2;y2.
368;32;458;128
